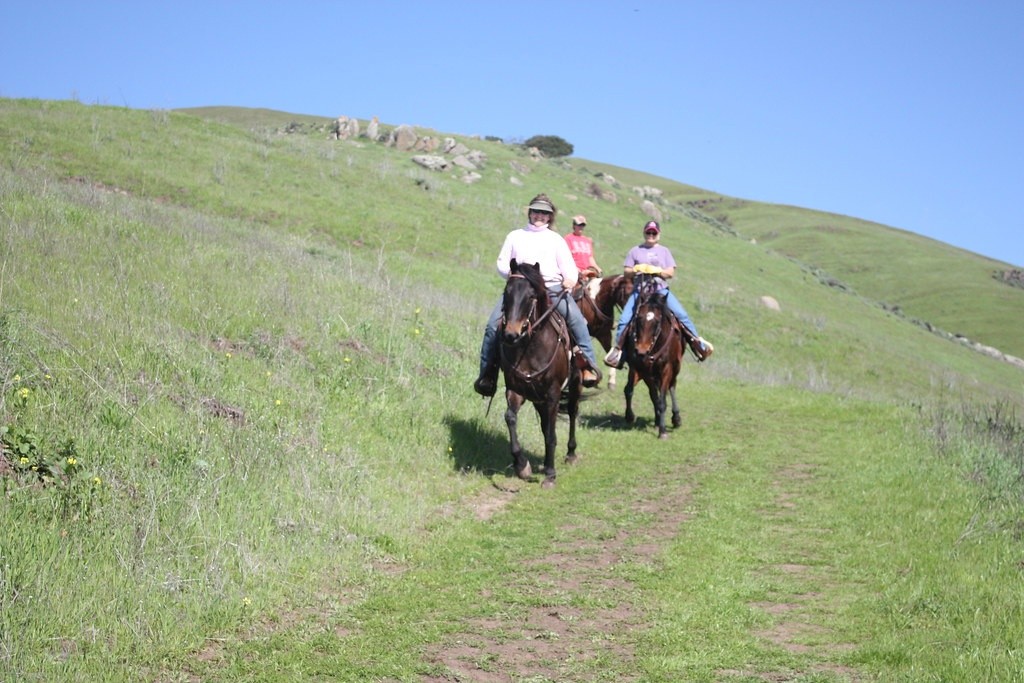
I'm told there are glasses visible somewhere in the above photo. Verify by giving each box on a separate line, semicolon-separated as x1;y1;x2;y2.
574;223;585;226
532;209;551;215
646;231;657;236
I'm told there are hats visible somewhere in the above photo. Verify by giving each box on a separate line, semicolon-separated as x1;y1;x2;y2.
644;221;660;233
573;215;587;225
528;195;554;212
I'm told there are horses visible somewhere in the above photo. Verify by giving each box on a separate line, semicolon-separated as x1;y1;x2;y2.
494;256;687;490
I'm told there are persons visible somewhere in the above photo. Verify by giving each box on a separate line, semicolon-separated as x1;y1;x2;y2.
474;193;599;397
563;215;604;298
603;221;713;370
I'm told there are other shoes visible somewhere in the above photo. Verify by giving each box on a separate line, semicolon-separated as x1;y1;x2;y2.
604;347;622;367
473;375;496;396
582;368;597;384
696;337;713;356
